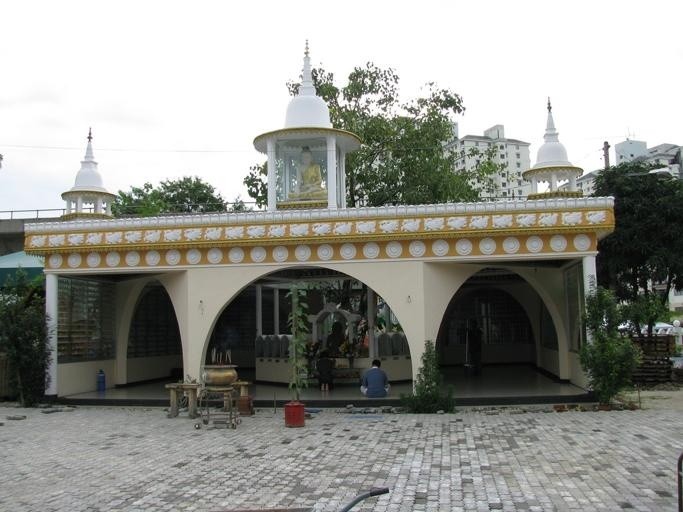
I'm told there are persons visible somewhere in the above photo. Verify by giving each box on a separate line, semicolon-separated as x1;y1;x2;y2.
312;351;336;391
358;359;391;398
467;320;482;371
286;145;328;200
